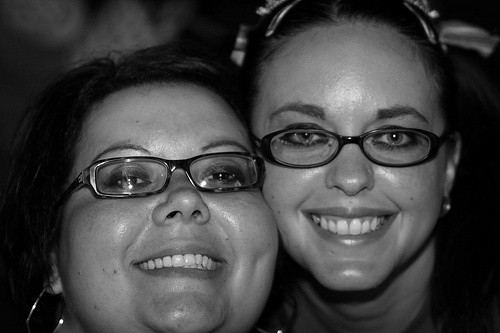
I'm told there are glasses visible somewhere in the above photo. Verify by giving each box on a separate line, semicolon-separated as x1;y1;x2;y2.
248;125;449;168
56;150;266;208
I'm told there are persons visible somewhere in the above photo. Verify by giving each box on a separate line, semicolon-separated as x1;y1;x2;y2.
0;43;280;333
228;0;500;333
